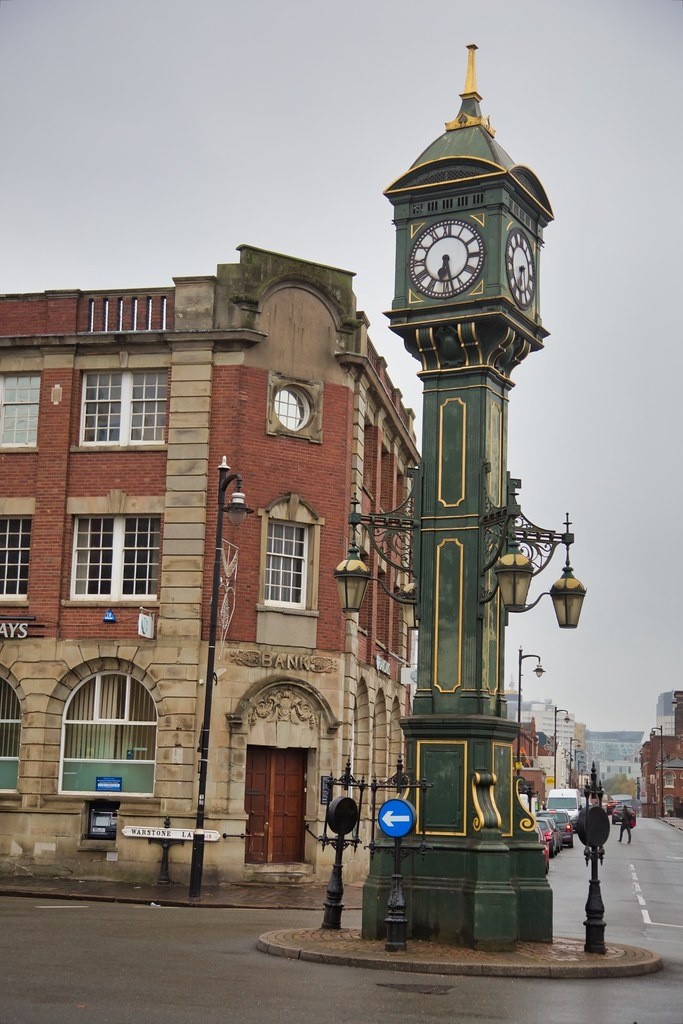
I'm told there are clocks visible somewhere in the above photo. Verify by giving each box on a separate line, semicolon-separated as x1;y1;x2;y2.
505;227;537;310
411;219;486;298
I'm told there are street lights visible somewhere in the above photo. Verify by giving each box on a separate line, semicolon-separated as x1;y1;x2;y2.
553;706;570;790
574;749;587;790
649;726;664;816
517;645;546;778
187;454;256;898
330;494;586;949
569;736;581;789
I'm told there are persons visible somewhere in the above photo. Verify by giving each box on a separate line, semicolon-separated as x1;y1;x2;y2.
618;805;631;844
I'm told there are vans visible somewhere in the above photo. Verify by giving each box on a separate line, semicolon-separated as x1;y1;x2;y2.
541;789;582;833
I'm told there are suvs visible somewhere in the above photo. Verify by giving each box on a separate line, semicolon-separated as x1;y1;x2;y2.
611;805;637;825
535;810;574;849
606;801;621;816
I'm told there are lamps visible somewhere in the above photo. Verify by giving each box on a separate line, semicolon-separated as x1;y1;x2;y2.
334;493;417;614
482;504;586;630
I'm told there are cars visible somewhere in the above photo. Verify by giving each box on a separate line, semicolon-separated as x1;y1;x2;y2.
548;818;563;853
533;817;555;857
